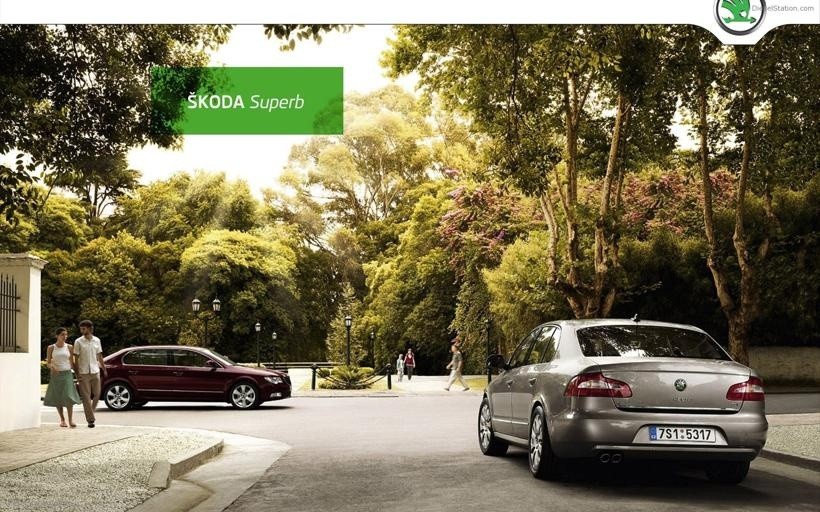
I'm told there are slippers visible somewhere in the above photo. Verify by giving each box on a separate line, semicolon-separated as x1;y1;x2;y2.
60;423;67;426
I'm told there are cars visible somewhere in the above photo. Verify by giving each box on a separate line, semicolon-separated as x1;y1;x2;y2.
478;313;769;485
73;345;292;411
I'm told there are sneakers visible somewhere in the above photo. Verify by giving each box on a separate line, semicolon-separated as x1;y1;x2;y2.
88;421;95;428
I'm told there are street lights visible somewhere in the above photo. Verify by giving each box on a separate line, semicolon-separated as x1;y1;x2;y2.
192;295;222;348
272;330;278;371
485;318;492;375
370;329;375;369
344;312;352;366
255;317;261;368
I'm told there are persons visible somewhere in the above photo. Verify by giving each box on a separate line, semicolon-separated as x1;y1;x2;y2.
45;326;77;429
405;348;416;379
72;318;108;428
396;353;405;382
444;344;469;391
